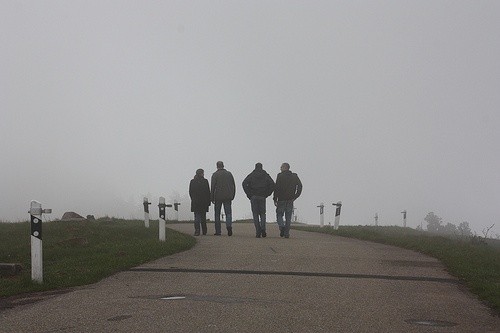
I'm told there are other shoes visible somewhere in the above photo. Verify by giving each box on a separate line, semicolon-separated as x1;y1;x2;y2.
285;236;289;238
203;232;206;235
256;232;261;237
262;233;266;237
195;232;200;236
280;227;285;237
228;232;232;236
215;233;221;236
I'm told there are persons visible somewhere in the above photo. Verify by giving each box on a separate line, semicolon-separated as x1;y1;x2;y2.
273;163;302;238
242;163;275;239
211;161;235;237
188;169;211;237
